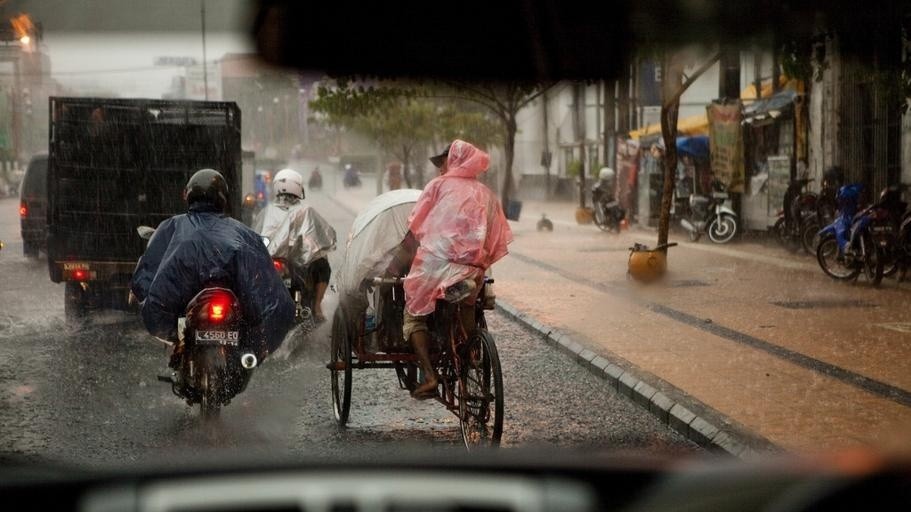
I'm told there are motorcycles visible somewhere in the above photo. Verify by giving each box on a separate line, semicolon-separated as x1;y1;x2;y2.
308;176;322;191
681;168;739;245
342;174;362;188
135;218;269;424
769;155;910;290
266;255;317;335
573;167;625;236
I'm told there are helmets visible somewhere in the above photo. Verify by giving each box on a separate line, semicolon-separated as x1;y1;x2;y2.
599;168;614;181
272;168;302;199
184;168;229;211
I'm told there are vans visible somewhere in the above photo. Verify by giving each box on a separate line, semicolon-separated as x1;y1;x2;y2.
17;149;48;262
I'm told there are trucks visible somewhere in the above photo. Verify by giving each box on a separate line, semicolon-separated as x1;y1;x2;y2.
45;91;244;326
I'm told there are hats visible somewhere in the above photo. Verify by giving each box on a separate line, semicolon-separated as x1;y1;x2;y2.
429;143;451;168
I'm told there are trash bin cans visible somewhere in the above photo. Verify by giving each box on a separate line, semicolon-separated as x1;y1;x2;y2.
506;199;522;221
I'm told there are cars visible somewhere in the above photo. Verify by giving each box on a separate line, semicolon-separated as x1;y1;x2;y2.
239;141;289;224
0;175;17;200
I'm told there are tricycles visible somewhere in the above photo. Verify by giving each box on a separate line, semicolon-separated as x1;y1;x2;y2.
325;263;513;458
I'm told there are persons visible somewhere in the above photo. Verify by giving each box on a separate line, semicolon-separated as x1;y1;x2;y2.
253;169;337;321
403;138;500;397
131;169;296;369
